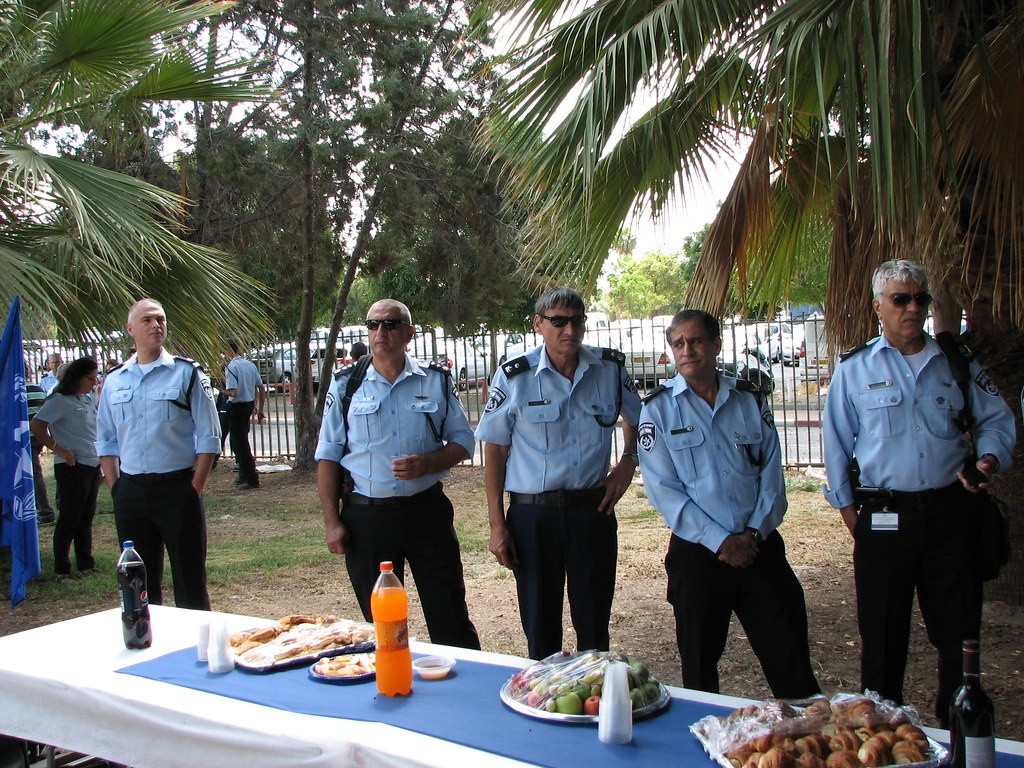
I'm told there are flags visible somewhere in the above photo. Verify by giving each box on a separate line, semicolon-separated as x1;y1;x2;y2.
0;298;41;609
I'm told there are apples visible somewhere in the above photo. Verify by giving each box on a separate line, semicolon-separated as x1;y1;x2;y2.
528;650;661;714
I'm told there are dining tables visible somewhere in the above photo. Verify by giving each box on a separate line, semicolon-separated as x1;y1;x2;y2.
0;600;1024;768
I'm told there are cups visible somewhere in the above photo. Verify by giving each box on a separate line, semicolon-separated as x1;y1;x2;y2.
197;622;210;661
598;662;632;745
206;626;235;673
390;456;407;477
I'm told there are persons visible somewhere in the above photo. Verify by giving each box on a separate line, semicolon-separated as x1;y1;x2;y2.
215;339;267;489
30;358;103;583
24;359;56;526
39;348;137;408
315;299;481;651
473;289;643;661
823;260;1016;726
637;310;822;708
350;342;368;361
212;367;239;472
94;298;222;610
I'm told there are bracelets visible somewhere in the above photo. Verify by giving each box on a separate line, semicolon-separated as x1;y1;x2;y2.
984;454;999;472
51;442;56;450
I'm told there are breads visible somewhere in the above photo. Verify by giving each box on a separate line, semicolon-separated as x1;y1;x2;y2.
702;699;930;768
230;614;375;662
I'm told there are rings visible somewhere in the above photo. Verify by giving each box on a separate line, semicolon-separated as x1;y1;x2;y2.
332;550;335;552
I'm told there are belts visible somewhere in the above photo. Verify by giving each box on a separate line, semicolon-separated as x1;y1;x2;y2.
348;492;427;507
508;488;604;506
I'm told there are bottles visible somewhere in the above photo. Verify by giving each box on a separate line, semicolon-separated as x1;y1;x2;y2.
370;561;413;695
116;540;152;649
950;639;996;768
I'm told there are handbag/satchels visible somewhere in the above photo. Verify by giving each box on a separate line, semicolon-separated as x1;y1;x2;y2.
976;490;1011;583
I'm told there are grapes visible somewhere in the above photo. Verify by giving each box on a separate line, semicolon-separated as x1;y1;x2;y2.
510;649;603;712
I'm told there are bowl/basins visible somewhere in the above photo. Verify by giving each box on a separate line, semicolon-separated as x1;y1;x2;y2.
411;655;456;680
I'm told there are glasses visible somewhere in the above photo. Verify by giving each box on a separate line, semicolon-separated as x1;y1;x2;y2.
541;313;587;328
83;374;96;382
877;292;933;309
366;317;410;330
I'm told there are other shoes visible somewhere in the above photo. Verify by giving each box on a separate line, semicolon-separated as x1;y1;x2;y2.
77;568;103;580
37;513;55;527
234;476;246;484
240;482;258;490
55;573;83;586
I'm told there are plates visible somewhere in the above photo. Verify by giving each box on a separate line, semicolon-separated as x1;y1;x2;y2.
308;653;375;681
500;681;672;723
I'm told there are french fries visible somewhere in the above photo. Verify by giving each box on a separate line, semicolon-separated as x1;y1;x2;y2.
314;653;375;676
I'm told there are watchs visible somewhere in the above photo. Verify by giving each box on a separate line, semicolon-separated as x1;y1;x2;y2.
751;530;762;543
623;451;639;469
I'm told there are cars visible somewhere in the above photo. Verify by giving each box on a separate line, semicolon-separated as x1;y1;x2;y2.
22;327;125;381
243;304;968;390
